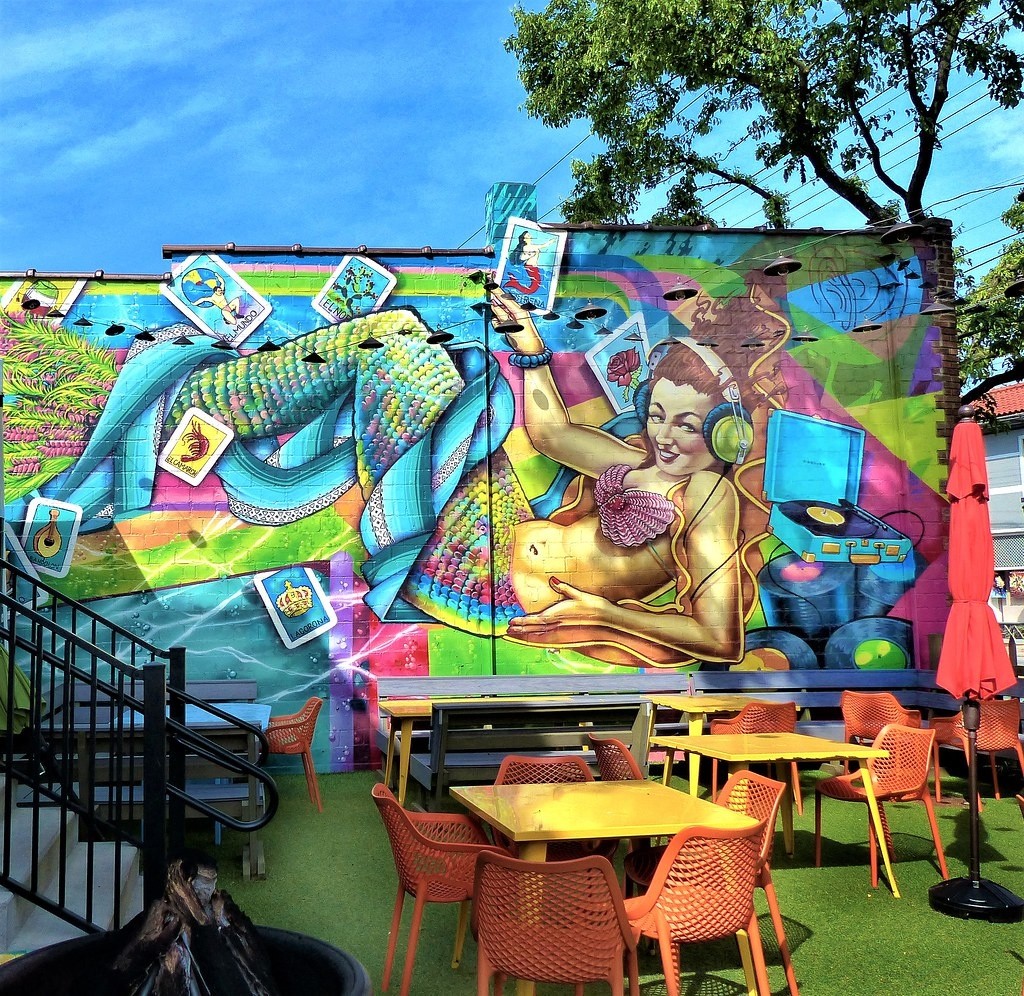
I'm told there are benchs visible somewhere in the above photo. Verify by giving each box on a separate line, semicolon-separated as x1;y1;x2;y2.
407;700;651;816
376;694;433;787
43;680;257;765
9;780;267;883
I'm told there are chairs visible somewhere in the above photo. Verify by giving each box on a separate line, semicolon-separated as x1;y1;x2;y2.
369;690;1024;996
257;697;325;812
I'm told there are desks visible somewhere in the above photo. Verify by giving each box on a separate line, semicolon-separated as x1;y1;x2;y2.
639;694;804;817
645;729;901;901
377;695;589;811
448;780;761;996
33;700;273;879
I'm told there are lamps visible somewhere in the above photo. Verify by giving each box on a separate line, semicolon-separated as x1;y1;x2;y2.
14;214;1024;365
